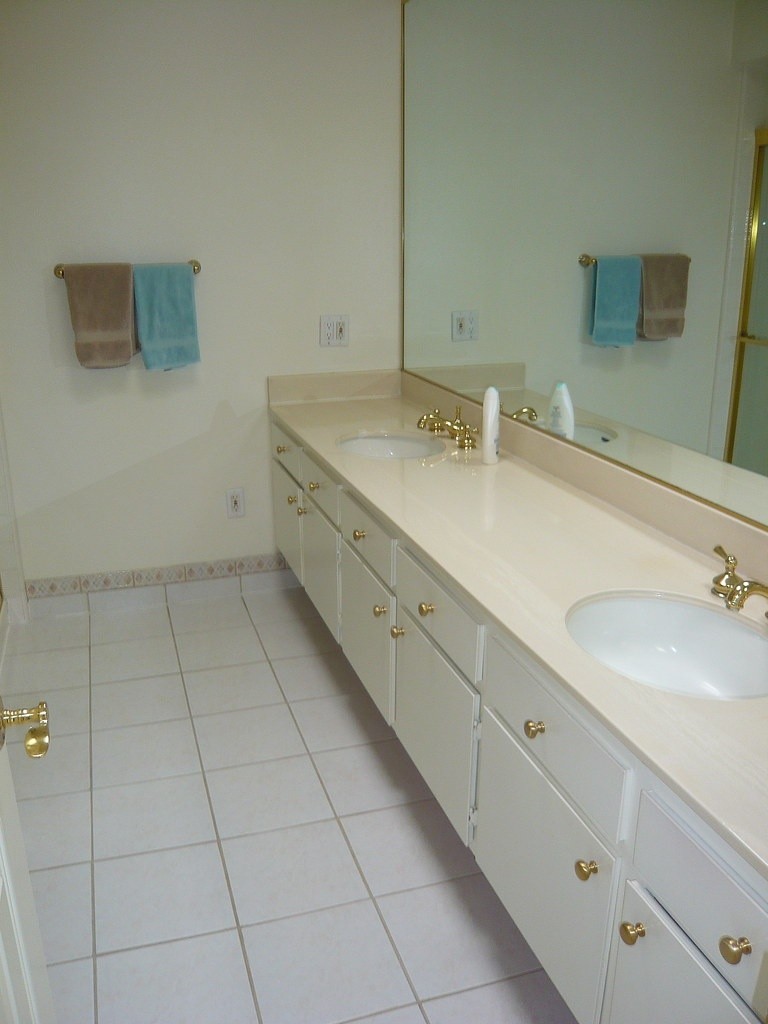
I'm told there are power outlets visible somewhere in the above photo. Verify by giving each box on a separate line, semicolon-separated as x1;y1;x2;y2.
320;315;348;347
226;488;244;518
451;310;478;341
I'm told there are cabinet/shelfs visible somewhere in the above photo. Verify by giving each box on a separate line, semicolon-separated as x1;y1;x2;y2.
337;489;485;850
472;626;768;1024
268;414;343;646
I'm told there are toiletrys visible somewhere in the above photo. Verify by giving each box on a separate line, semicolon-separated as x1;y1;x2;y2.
546;382;575;442
481;386;501;465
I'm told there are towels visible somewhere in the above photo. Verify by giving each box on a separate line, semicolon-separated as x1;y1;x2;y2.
587;254;642;347
636;253;692;342
132;263;201;372
63;262;141;369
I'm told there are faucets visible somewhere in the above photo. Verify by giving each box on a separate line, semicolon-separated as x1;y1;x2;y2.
725;581;768;618
426;405;465;440
511;406;537;421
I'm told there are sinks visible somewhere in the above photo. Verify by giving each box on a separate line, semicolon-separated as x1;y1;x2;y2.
564;589;768;703
335;428;447;460
533;420;618;445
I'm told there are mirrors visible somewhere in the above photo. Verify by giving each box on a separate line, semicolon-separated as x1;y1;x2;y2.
398;0;768;532
